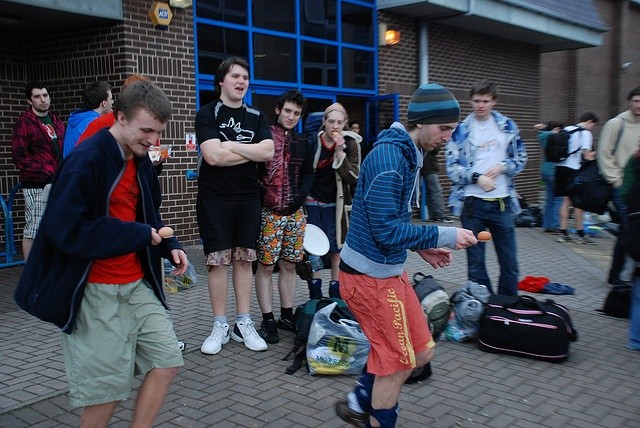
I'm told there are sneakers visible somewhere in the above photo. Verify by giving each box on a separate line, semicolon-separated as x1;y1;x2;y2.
278;314;296;330
260;316;280;344
556;231;577;241
334;389;370;427
231;317;268;352
200;320;230;354
434;216;455;223
574;233;598;244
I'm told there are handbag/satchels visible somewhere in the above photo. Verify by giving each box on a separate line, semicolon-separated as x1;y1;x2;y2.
605;276;633;318
295;250;313;280
515;206;542;228
567;157;614;215
404;362;433;383
474;293;578;363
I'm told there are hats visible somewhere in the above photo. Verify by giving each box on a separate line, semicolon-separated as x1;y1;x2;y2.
405;82;460;125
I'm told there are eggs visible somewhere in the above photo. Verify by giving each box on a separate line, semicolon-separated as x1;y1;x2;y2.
158;227;174;237
478;231;491;240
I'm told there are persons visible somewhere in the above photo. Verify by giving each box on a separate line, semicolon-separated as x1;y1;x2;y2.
10;79;67;263
255;88;314;343
532;119;564;233
420;143;455;224
301;101;361;300
74;74;185;352
13;77;186;427
443;78;529;297
595;85;640;285
349;120;361;134
334;81;479;428
618;146;640;351
62;80;115;162
553;112;600;245
194;53;276;355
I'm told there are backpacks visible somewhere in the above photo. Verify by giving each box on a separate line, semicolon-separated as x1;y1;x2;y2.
543;125;585;162
412;271;451;342
282;296;356;374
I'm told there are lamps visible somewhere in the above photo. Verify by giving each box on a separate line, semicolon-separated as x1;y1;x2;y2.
378;23;401;47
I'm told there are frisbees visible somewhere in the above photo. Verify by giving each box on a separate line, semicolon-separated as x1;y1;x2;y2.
303;223;330;256
